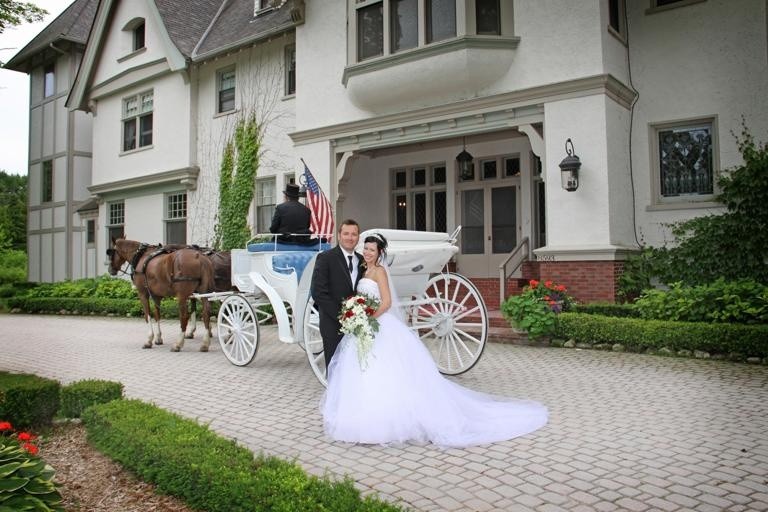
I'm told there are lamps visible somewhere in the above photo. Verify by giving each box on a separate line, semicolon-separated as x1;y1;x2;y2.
559;137;581;192
297;173;307;197
456;136;473;179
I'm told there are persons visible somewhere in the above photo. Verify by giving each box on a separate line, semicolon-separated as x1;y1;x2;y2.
269;182;312;244
319;233;551;448
310;219;367;387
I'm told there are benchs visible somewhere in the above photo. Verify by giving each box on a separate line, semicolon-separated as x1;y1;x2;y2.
246;229;449;286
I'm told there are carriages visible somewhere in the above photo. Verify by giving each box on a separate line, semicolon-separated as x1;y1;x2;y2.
107;225;490;388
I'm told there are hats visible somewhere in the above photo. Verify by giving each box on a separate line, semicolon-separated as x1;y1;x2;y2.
283;183;299;197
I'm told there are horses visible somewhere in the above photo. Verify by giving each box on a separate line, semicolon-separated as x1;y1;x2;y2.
158;242;245;343
107;235;216;352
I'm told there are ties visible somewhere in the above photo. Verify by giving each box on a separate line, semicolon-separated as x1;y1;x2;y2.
348;256;353;273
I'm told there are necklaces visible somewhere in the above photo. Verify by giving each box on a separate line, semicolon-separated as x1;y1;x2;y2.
363;265;375;277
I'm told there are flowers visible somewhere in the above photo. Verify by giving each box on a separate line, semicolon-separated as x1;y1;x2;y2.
337;290;382;373
522;280;573;313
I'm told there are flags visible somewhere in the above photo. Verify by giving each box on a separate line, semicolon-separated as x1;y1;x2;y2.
305;165;335;245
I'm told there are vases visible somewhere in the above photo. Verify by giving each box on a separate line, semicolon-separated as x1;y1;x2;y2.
549;300;564;312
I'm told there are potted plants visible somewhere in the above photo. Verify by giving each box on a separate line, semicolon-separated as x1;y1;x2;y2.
503;291;555;341
